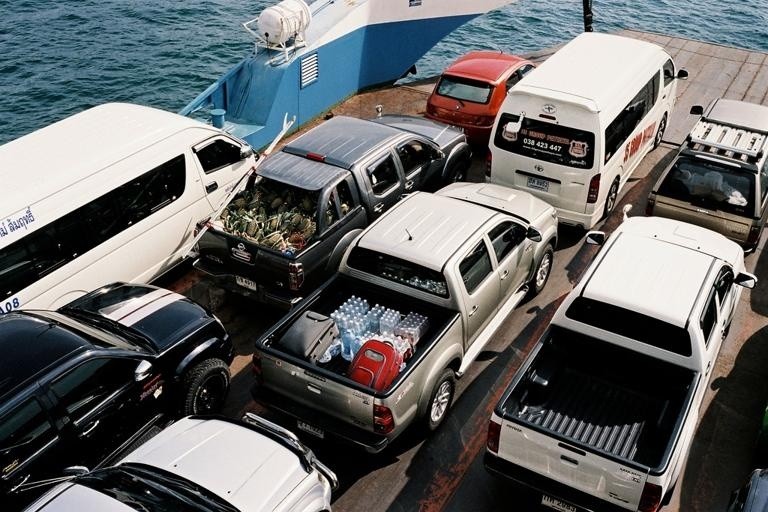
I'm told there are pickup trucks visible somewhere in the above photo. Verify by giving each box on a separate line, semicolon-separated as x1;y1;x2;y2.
251;177;557;452
192;114;474;306
483;214;759;503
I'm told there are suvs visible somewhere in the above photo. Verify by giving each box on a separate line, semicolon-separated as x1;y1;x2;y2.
1;280;234;502
649;94;764;254
24;412;340;512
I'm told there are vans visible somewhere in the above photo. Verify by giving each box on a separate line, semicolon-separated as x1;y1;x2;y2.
0;103;259;315
487;32;689;231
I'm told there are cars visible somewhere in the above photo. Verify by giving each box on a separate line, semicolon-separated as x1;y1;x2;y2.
426;50;535;131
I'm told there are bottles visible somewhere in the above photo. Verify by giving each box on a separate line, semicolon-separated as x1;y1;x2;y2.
366;303;385;335
380;308;399;338
341;331;354;360
383;270;447;295
355;330;379;355
383;335;414;369
330;295;369;334
397;311;427;347
